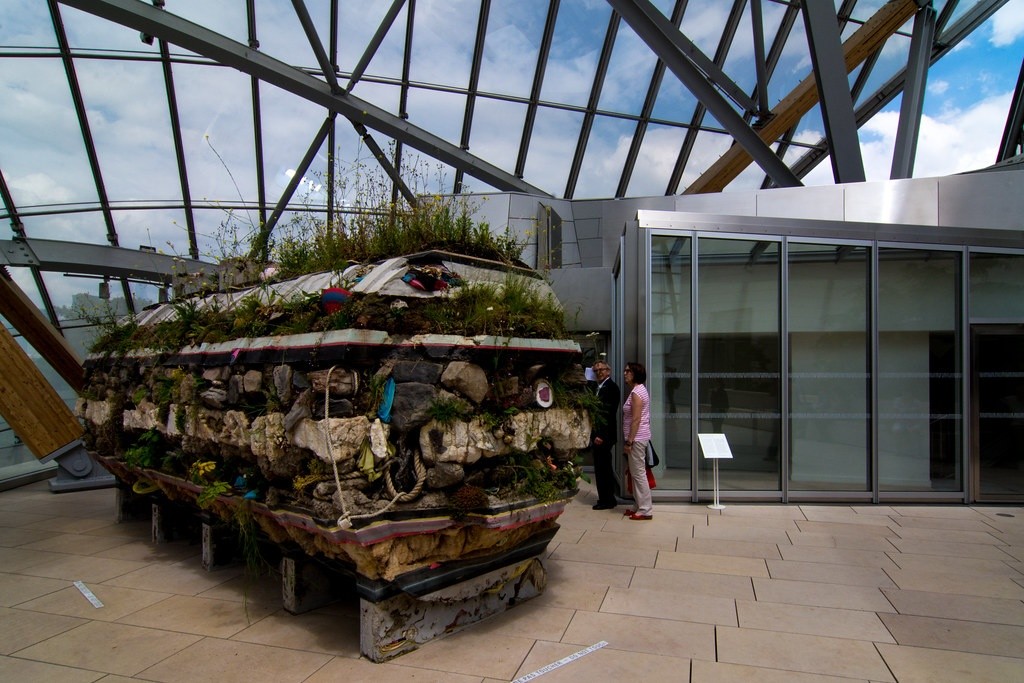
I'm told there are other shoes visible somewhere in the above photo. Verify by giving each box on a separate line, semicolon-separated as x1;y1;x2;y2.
624;510;636;516
629;513;652;520
593;499;618;510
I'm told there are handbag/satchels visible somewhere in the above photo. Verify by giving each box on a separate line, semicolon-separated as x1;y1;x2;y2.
625;463;656;495
645;439;659;468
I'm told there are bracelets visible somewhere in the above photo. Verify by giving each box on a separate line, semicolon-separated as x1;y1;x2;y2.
625;440;632;446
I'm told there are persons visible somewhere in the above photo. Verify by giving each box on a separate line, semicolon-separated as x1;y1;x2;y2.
591;360;620;510
621;362;653;519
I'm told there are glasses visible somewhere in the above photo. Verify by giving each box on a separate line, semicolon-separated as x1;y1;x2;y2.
594;367;609;373
623;369;631;373
